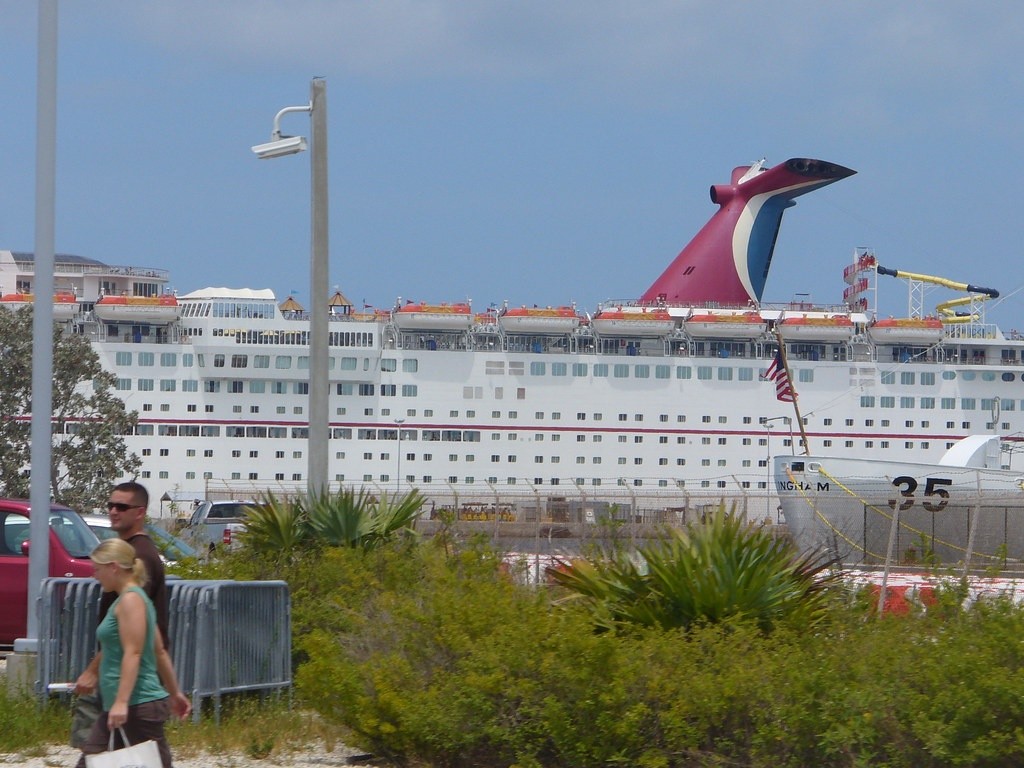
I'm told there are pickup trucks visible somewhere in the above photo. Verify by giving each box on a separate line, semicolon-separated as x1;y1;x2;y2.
178;499;270;565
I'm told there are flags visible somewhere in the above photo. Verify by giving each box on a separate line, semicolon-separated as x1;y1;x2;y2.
290;289;300;295
759;343;798;402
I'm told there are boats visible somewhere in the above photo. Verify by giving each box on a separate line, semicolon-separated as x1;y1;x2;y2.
774;433;1023;575
866;319;943;350
392;298;473;331
590;304;671;341
499;308;581;337
681;308;769;342
2;291;82;328
93;287;177;326
777;314;857;342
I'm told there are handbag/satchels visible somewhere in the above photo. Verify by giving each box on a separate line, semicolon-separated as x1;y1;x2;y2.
84;723;165;768
70;692;101;751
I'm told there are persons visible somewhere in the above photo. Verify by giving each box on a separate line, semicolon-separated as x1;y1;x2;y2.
67;481;169;748
82;536;191;767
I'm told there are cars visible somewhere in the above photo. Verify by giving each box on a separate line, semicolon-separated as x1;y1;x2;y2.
0;499;101;645
4;513;222;575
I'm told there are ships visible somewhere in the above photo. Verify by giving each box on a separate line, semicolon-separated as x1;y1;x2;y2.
0;156;1024;518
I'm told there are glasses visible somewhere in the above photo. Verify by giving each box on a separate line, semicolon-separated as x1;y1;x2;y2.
106;501;143;512
